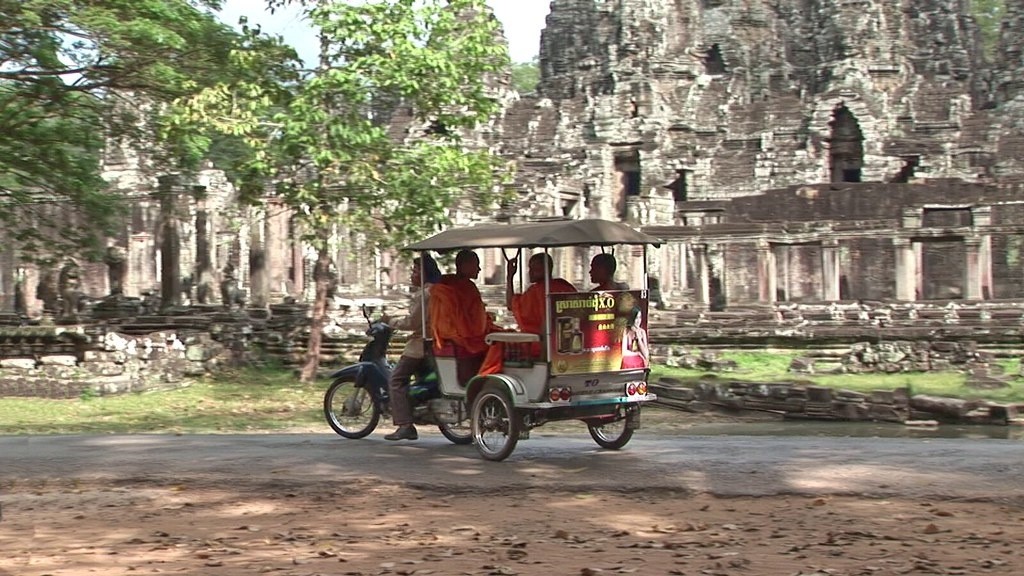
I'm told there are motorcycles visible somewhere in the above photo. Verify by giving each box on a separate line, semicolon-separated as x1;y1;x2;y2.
320;216;668;464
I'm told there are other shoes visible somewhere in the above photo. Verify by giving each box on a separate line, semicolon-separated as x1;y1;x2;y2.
384;427;418;440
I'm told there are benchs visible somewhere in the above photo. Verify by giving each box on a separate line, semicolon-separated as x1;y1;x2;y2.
432;332;547;369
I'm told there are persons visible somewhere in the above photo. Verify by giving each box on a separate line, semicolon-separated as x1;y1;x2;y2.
506;254;578;358
588;253;629;292
428;249;506;377
618;305;650;369
380;256;442;441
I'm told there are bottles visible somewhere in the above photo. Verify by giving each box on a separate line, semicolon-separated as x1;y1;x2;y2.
571;318;583;354
631;337;638;352
